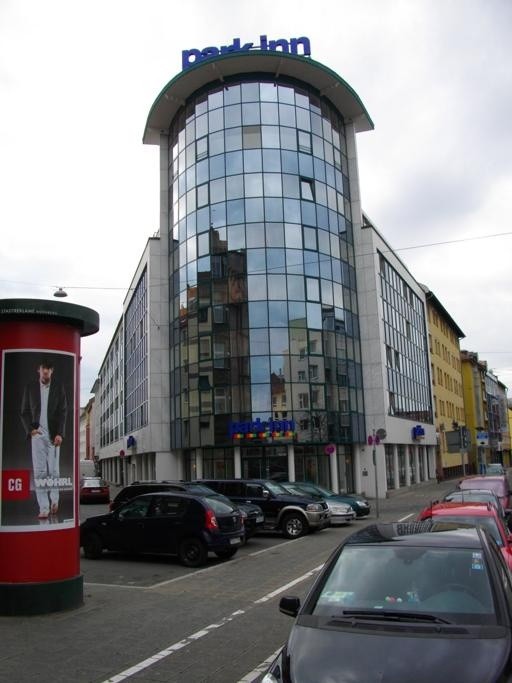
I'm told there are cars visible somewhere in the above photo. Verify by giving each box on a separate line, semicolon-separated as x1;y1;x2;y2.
456;473;511;517
78;471;332;569
443;487;506;519
261;521;512;682
417;500;511;565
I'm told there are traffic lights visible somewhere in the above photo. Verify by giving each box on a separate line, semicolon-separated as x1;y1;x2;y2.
462;427;469;447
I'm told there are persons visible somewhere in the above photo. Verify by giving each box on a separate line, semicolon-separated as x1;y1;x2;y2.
18;355;67;520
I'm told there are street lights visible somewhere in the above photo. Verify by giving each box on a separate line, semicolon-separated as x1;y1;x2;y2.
369;425;387;520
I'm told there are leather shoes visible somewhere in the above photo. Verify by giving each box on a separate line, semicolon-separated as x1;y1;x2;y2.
38;512;48;518
51;502;58;514
